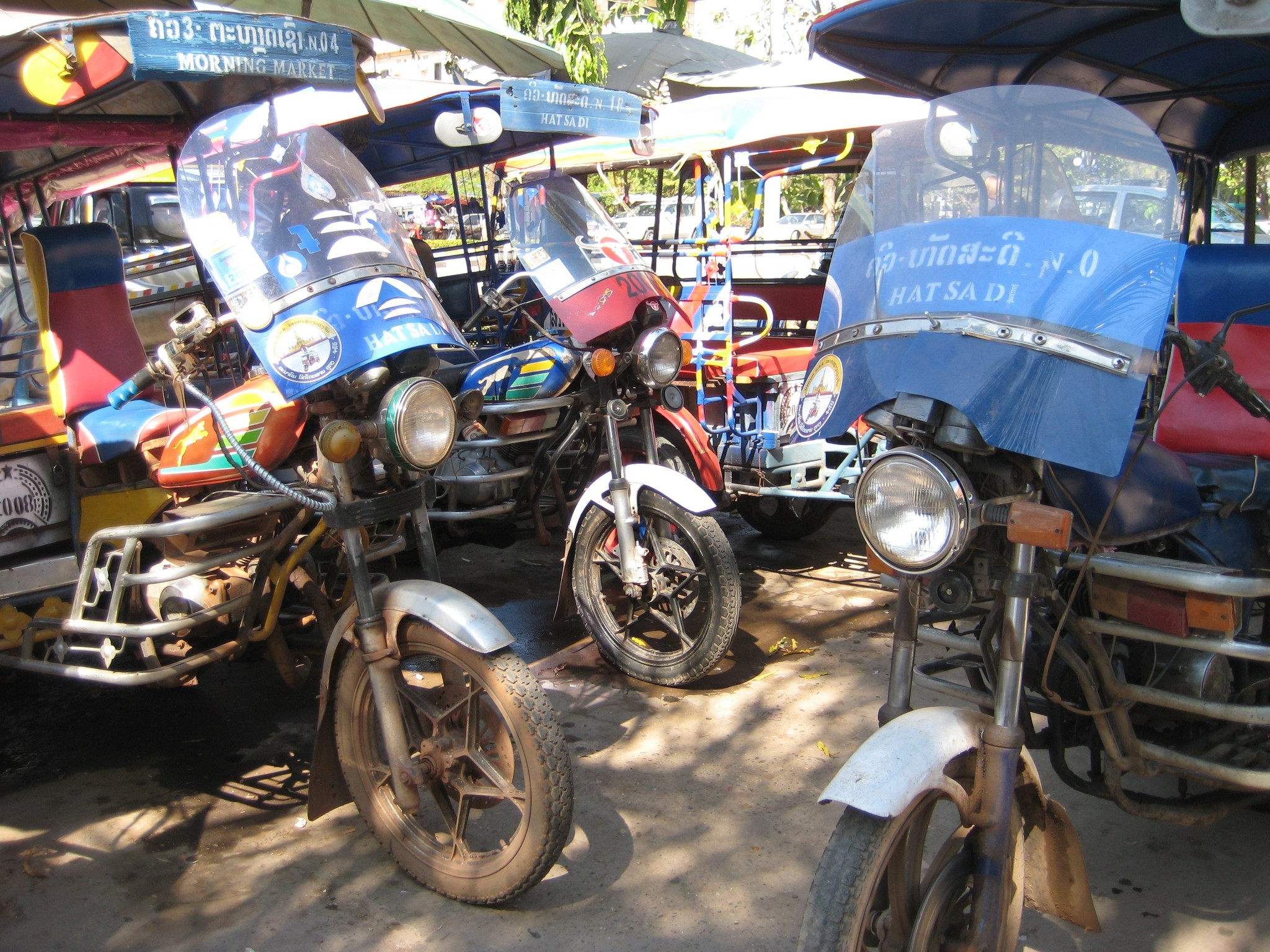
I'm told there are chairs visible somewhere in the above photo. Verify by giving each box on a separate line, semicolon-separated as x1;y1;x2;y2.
19;219;205;461
1153;242;1270;513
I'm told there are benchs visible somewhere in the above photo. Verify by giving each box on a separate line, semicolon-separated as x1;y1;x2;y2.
660;277;830;379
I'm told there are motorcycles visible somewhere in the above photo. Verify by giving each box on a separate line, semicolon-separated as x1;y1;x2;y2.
0;70;964;688
0;6;579;910
781;0;1270;952
